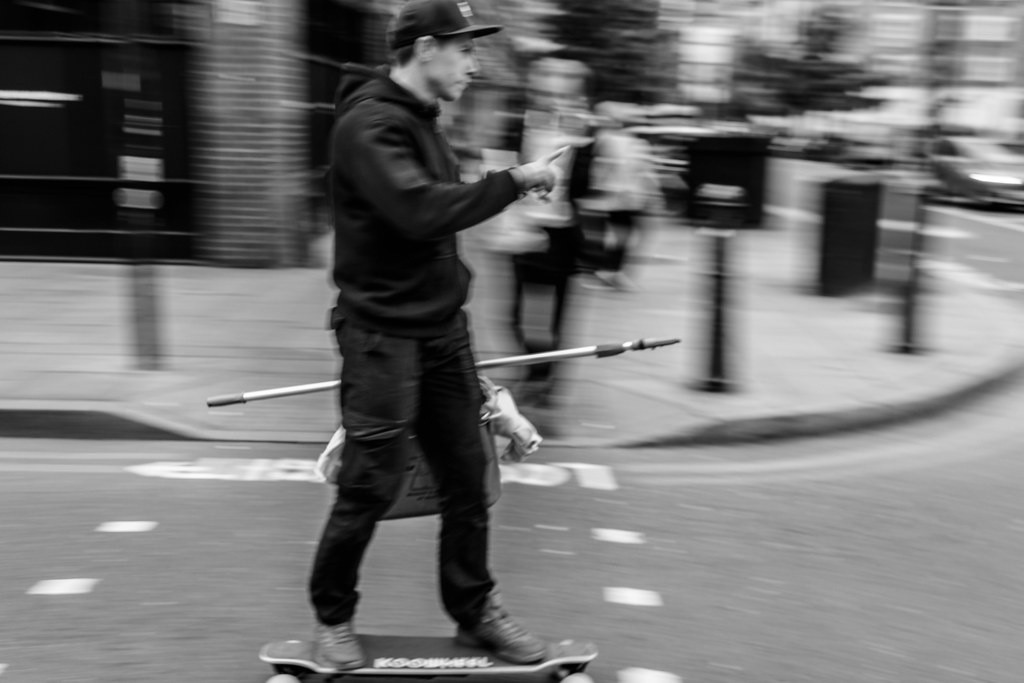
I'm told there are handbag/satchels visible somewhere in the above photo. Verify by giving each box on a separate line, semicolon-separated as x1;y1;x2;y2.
316;376;543;521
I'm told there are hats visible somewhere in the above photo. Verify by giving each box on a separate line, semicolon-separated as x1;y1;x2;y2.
386;0;504;51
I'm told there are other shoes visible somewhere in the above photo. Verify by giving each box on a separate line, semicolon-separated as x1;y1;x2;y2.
312;622;366;670
456;587;546;662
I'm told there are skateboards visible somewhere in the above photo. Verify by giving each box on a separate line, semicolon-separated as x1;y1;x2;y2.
259;635;600;682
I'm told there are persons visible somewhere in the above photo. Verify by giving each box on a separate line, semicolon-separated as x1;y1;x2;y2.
307;0;570;670
